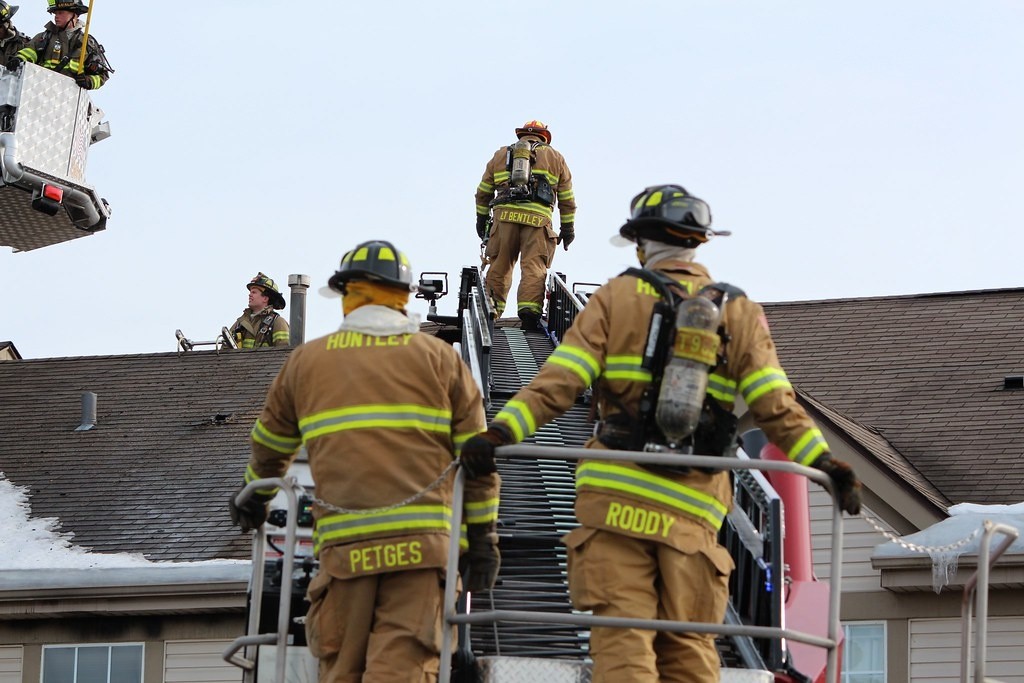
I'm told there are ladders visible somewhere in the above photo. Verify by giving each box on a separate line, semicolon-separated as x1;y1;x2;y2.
175;326;239;350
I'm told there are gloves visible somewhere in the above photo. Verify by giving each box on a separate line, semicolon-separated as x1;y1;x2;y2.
557;228;578;252
457;524;501;593
475;215;487;240
6;56;21;72
75;73;89;88
458;425;510;481
229;482;274;534
808;454;861;519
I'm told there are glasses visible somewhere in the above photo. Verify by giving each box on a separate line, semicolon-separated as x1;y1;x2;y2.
657;194;712;228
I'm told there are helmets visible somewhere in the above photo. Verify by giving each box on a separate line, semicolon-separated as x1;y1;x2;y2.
326;242;436;295
514;120;552;147
245;272;285;311
47;0;89;14
0;0;19;24
620;183;713;242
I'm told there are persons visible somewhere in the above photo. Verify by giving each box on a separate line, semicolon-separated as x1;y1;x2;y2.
221;272;290;350
460;183;861;682
6;0;115;90
474;120;576;324
230;240;501;683
0;0;33;132
